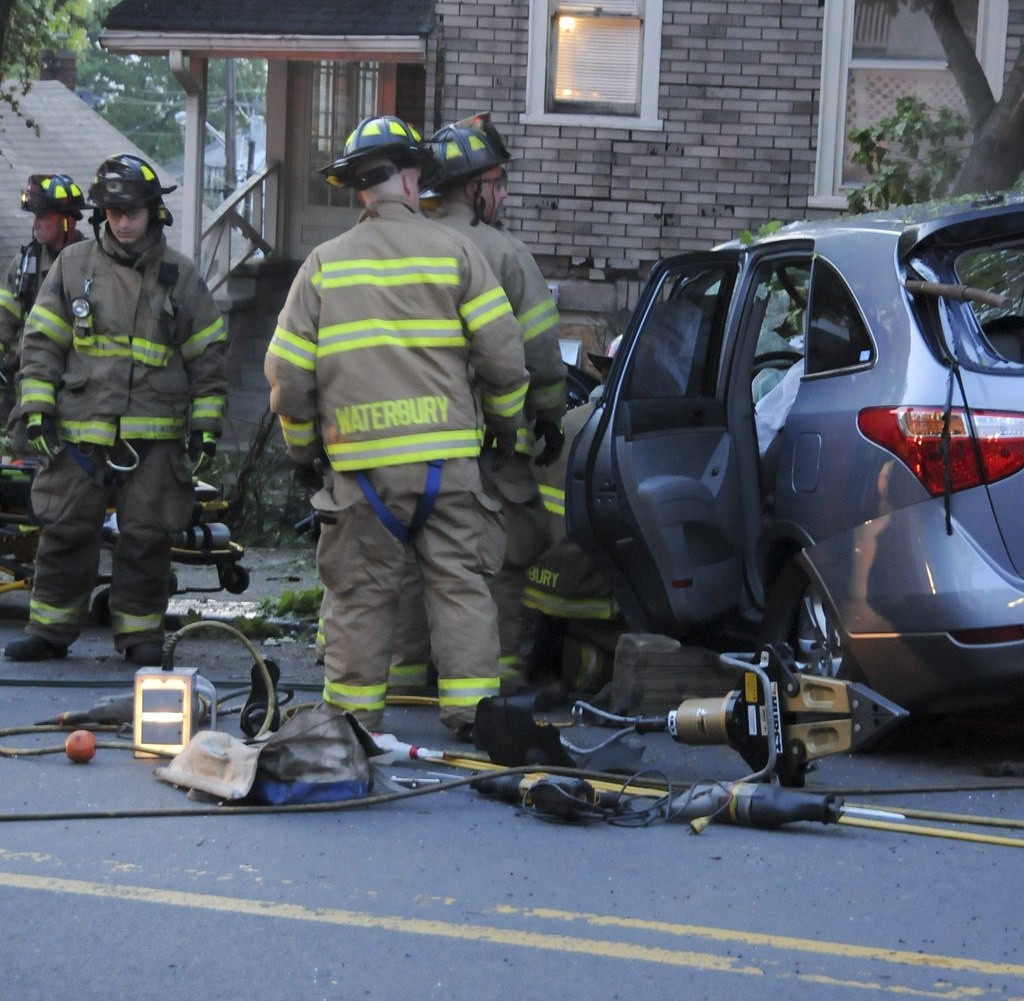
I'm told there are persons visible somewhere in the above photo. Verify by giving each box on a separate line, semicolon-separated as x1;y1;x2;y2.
0;155;231;666
264;111;567;746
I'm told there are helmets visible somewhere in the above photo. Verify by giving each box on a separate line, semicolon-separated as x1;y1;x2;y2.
317;115;435;189
21;173;100;213
89;153;177;208
422;124;517;189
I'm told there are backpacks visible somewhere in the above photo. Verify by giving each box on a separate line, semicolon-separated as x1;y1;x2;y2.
517;536;621;692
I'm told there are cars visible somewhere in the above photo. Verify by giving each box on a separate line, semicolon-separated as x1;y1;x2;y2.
565;194;1024;738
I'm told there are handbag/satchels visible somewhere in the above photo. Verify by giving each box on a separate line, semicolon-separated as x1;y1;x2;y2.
252;702;393;805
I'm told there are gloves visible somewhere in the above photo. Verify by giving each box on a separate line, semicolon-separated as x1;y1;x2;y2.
531;403;567;467
482;413;519;472
284;442;332;491
188;430;216;475
25;413;56;459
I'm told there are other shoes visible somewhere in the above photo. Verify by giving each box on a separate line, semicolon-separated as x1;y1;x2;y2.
125;641;165;664
387;687;440;707
454;724;474;743
4;633;69;659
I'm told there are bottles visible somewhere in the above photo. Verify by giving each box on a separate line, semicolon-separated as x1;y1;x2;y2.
366;731;444;766
